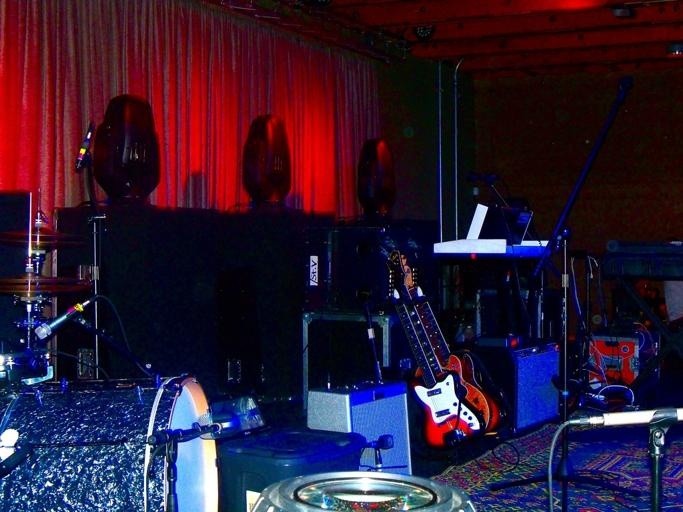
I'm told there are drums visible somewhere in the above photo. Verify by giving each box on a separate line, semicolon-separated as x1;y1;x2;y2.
0;374;220;512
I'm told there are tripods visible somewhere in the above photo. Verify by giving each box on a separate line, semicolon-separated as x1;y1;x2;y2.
487;72;640;511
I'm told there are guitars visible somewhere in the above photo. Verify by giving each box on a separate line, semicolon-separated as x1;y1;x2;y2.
385;247;502;449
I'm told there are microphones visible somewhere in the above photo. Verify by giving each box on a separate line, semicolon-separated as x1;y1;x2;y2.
75;123;95;169
364;432;396;452
603;408;682;427
35;298;92;340
148;422;221;446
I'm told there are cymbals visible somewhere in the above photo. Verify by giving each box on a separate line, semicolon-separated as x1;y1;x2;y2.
1;272;91;293
10;226;65;249
